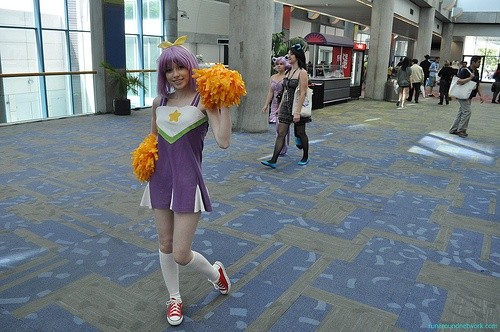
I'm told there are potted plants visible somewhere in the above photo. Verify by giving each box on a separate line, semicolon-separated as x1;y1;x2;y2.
98;61;149;115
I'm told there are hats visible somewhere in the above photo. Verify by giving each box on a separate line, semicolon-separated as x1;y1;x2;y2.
424;55;431;59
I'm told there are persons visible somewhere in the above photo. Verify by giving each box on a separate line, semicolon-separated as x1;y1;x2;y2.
132;36;247;326
261;44;311;168
363;55;500;137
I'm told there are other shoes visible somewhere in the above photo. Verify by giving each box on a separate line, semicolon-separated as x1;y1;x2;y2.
459;132;468;137
449;129;458;135
438;102;443;105
279;147;288;156
415;100;419;103
445;103;449;105
406;99;411;102
428;94;435;97
491;99;498;104
396;100;400;106
297;158;310;165
261;161;276;169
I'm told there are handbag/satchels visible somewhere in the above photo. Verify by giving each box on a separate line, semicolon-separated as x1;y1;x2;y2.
276;89;284;103
491;84;496;92
448;68;477;101
292;70;313;117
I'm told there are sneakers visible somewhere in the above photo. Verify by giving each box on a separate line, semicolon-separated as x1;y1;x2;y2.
207;261;231;295
166;298;184;326
